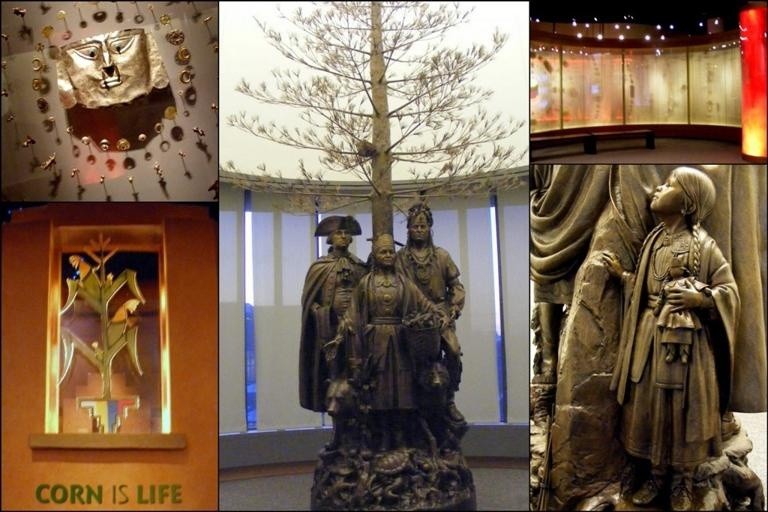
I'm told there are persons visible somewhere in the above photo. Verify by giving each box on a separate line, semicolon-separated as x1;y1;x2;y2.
321;233;450;434
599;166;743;510
386;199;466;423
295;214;372;449
651;257;714;368
